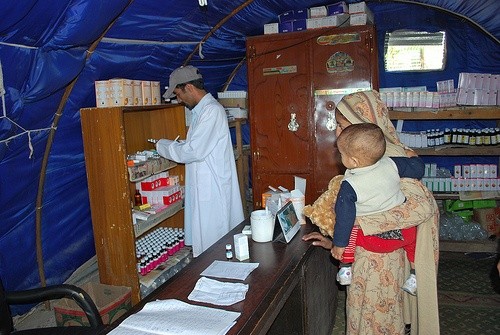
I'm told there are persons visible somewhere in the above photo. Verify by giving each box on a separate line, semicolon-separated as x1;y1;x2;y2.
302;90;441;335
330;123;419;297
153;65;244;257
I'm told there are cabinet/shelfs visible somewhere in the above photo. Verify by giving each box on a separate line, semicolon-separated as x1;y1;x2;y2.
245;23;379;211
389;108;500;252
96;212;339;335
80;103;185;303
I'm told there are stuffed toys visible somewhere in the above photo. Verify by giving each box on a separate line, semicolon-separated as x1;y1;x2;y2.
302;175;345;240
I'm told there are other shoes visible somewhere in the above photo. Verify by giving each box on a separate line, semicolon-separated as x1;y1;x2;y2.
401;276;417;296
335;266;352;286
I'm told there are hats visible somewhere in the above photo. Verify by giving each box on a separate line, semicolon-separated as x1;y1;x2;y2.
162;64;202;99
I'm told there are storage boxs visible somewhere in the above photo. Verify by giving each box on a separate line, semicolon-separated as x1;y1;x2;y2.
379;72;500;108
54;282;132;327
443;200;499;237
262;174;306;224
264;2;374;34
95;80;160;108
422;163;500;192
218;90;248;118
136;172;184;204
396;132;427;147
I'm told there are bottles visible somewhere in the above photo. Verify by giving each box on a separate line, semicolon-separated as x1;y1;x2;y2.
135;190;141;206
225;244;233;260
250;210;274;243
425;127;500;147
134;227;184;275
